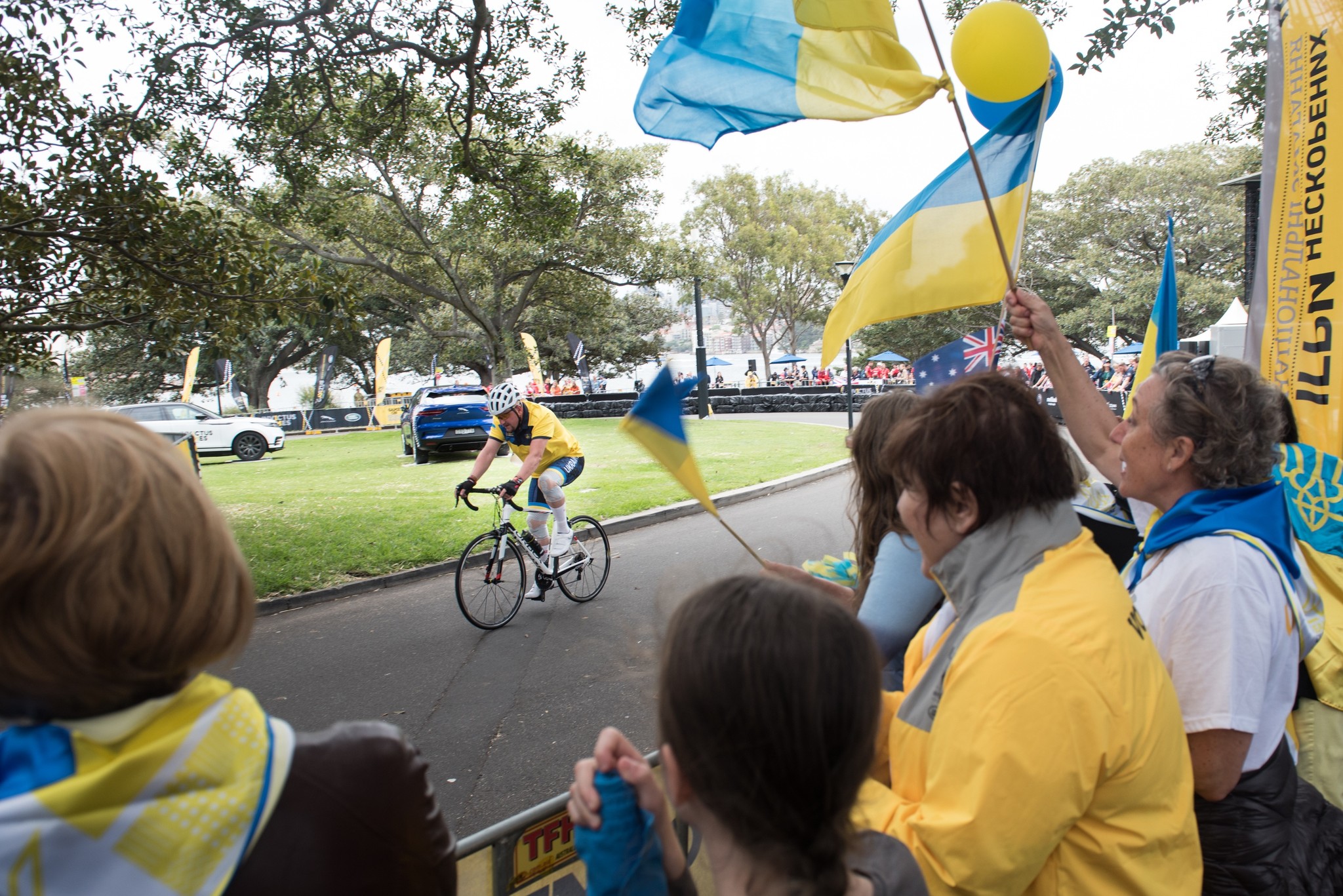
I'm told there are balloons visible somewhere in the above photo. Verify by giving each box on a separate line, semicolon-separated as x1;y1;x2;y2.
950;0;1063;136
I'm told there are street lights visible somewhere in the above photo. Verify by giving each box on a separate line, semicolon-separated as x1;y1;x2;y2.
834;258;855;431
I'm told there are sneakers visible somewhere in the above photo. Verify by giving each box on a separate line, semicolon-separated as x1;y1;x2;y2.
550;528;574;558
524;584;541;599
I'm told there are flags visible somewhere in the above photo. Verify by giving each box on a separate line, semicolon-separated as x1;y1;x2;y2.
819;88;1044;369
632;1;955;150
1121;235;1177;419
621;365;717;518
908;325;999;394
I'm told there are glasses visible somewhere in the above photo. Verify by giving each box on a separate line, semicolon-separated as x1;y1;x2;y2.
498;405;517;421
1182;354;1218;404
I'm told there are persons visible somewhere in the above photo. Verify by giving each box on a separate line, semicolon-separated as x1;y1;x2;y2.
563;575;933;896
1005;286;1342;896
864;361;915;384
765;393;945;692
457;384;585;599
354;390;365;406
672;371;724;389
766;365;834;386
526;378;582;396
0;403;460;895
846;371;1204;895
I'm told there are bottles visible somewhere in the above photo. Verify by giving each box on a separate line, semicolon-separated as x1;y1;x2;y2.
522;529;544;556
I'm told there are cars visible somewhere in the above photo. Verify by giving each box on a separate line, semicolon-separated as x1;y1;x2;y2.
399;384;510;465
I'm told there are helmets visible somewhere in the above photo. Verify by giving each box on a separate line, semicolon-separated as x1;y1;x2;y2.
487;382;522;416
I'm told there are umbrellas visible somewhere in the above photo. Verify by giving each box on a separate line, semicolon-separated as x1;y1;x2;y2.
866;349;911;362
768;353;807;373
695;357;732;383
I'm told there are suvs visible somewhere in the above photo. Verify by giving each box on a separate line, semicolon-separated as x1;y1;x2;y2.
100;401;286;462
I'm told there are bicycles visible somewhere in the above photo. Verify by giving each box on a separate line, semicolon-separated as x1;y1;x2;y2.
453;483;611;631
